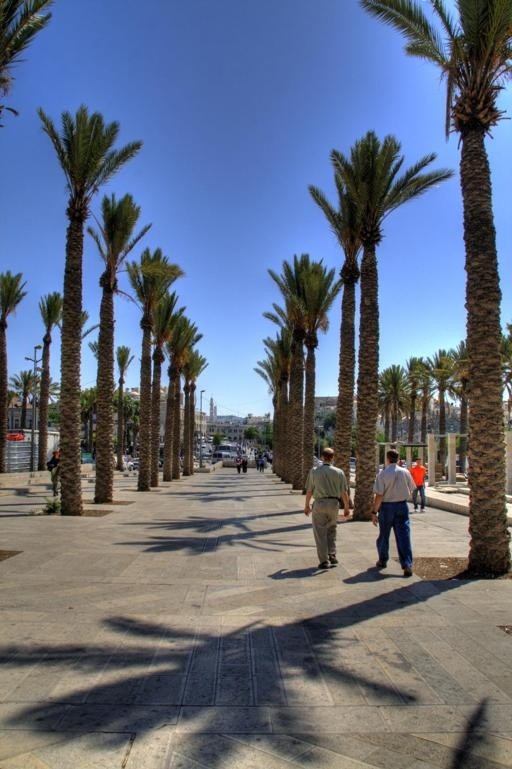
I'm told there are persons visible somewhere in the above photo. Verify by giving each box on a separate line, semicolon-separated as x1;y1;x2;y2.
47;450;60;497
372;450;417;576
410;458;427;513
304;448;349;568
399;459;403;467
316;454;324;468
235;447;274;473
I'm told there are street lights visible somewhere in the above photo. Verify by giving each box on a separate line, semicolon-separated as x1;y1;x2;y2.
198;389;207;468
316;425;325;459
25;345;42;473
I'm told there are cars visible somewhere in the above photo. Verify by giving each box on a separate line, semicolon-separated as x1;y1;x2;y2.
80;433;241;472
350;456;357;465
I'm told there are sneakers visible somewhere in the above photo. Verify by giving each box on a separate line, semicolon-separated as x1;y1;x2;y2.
329;555;338;564
404;567;413;575
317;561;328;568
376;561;387;567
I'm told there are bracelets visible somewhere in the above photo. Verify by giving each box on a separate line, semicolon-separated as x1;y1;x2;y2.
371;511;378;514
